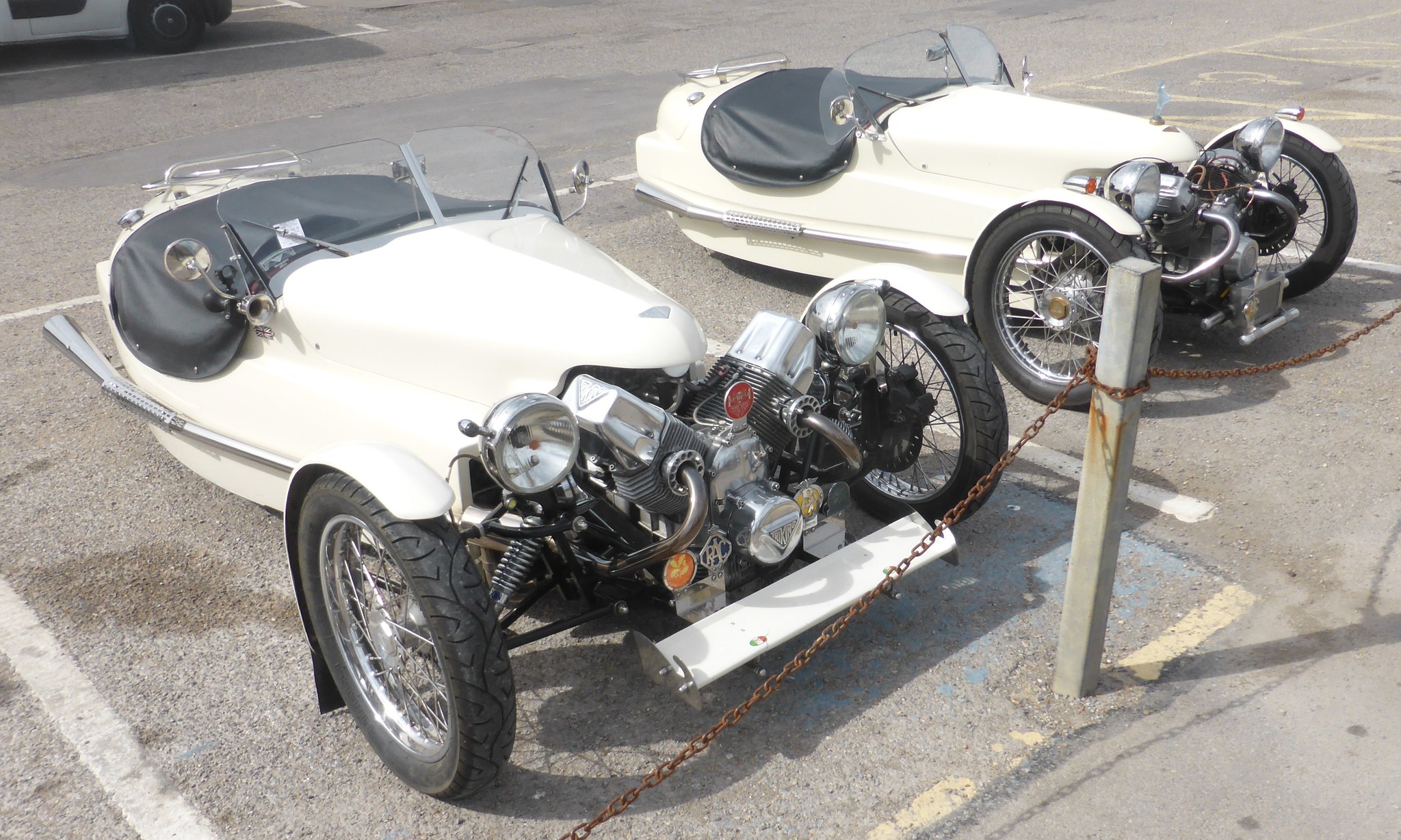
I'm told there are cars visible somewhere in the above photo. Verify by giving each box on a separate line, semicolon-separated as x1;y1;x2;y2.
632;21;1359;414
1;0;240;57
43;144;1015;804
